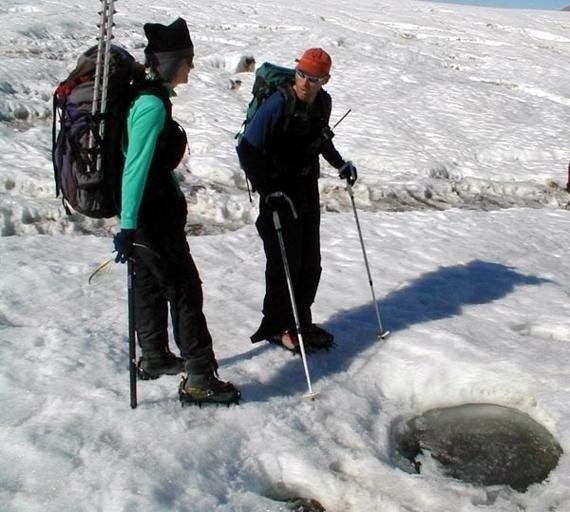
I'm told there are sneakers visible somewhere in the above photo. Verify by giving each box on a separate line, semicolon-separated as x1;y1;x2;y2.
178;371;241;408
136;351;186;379
262;319;334;354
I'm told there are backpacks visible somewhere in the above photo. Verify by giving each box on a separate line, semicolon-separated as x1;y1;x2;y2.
235;62;335;169
51;43;173;219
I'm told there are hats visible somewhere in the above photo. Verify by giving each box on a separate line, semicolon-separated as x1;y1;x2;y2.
296;48;332;79
143;18;193;54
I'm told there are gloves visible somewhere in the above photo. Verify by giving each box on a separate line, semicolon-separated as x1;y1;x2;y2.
267;195;287;210
112;229;136;263
338;165;357;186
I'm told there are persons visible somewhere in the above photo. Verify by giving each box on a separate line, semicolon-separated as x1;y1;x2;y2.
237;48;357;349
114;15;241;403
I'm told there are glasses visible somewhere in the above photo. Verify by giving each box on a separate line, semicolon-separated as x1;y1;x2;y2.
296;68;319;83
